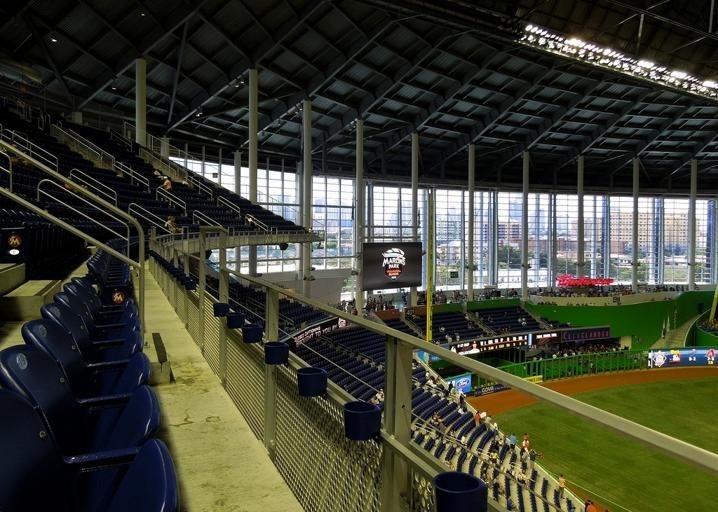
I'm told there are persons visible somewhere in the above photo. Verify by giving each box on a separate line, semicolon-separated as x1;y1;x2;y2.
165;215;181;233
154;169;163;181
558;474;566;499
583;498;600;512
160;175;171;201
372;310;633;482
66;174;79;192
703;318;718;330
182;177;189;185
338;281;689;315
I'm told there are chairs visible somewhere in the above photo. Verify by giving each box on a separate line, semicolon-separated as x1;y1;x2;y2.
0;103;576;512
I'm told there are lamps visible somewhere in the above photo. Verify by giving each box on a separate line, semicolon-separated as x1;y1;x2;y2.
512;19;718;103
233;73;245;88
111;79;117;90
